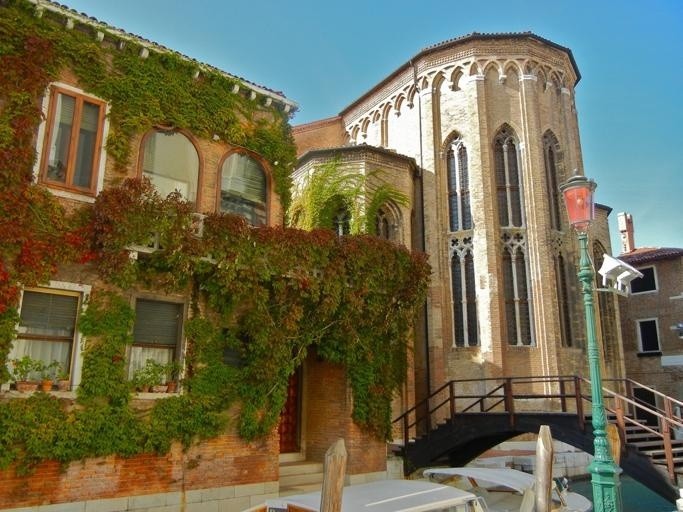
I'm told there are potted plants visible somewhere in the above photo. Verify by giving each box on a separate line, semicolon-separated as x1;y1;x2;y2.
128;356;184;394
11;355;70;394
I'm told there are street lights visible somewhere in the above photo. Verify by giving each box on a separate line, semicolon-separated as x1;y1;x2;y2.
558;167;624;512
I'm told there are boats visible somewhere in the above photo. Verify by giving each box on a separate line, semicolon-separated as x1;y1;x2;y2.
242;468;594;512
